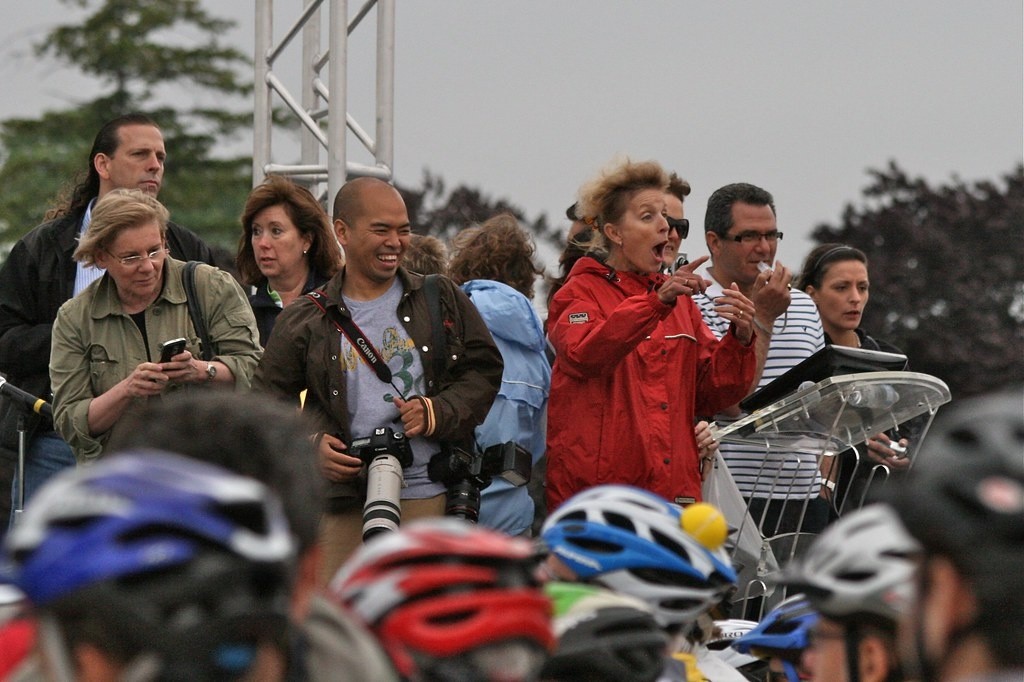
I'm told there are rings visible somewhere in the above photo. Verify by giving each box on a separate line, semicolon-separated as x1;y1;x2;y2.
788;284;792;290
738;310;743;319
683;278;689;286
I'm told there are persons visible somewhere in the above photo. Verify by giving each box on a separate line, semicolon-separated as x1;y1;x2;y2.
0;110;219;533
0;162;1024;682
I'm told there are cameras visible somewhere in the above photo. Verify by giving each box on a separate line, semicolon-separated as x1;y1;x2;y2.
668;252;689;274
876;440;909;461
427;441;532;528
350;426;414;544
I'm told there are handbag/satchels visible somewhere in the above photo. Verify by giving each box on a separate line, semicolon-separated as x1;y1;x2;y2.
700;439;784;599
0;373;49;454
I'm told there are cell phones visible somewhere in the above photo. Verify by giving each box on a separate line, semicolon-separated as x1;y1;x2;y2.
160;338;186;370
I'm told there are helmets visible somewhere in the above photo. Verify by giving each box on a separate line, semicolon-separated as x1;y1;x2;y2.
903;386;1024;570
289;578;400;682
332;518;555;682
736;592;822;662
700;618;772;672
539;488;739;623
1;451;298;658
537;574;670;682
794;499;924;622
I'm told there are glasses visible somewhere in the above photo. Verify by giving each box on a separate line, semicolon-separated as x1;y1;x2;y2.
99;237;171;266
666;216;689;239
722;230;783;243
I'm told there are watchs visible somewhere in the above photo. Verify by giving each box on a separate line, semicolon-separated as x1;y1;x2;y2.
206;361;217;383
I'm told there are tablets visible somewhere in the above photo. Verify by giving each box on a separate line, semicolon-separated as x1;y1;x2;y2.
738;345;910;414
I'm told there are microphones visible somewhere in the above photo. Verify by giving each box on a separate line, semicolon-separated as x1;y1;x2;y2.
0;375;54;417
